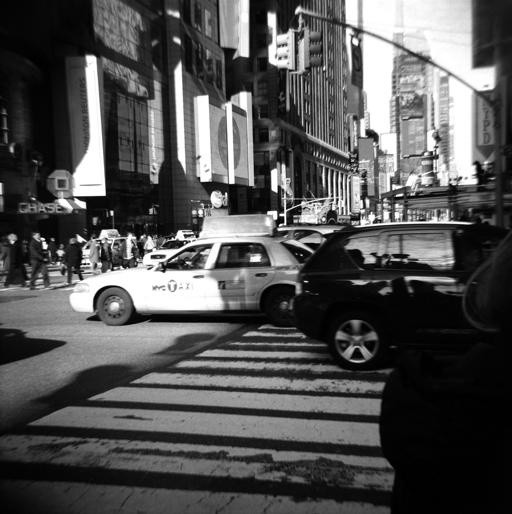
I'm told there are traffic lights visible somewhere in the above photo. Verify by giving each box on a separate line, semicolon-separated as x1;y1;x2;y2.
275;26;296;74
303;27;326;73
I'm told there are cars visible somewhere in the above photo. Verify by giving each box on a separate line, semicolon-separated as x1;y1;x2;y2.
75;227;198;271
67;213;315;326
270;222;348;252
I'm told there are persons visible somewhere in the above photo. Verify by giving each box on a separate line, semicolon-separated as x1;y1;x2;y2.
0;230;176;291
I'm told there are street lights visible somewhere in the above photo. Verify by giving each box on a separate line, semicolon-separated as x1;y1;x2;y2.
109;209;115;228
365;128;380;206
152;204;160;228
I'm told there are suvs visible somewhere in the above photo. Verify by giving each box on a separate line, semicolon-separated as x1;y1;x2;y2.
290;220;512;372
